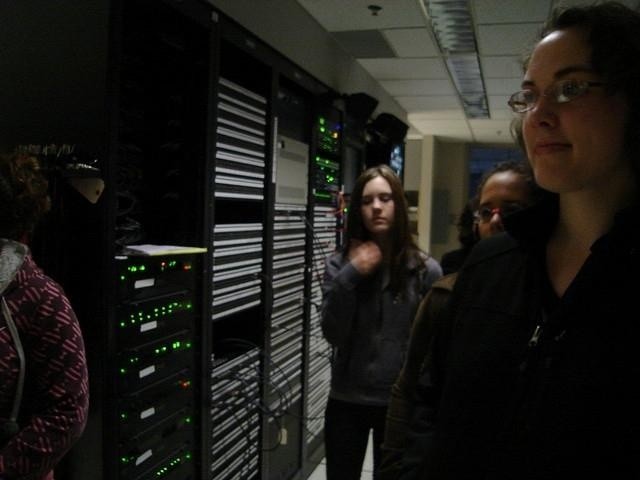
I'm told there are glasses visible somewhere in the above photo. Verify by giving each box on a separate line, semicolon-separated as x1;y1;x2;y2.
472;204;526;225
508;78;621;115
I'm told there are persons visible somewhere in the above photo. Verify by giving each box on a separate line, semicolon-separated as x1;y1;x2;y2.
319;162;443;480
417;0;640;480
377;156;540;479
1;174;91;480
